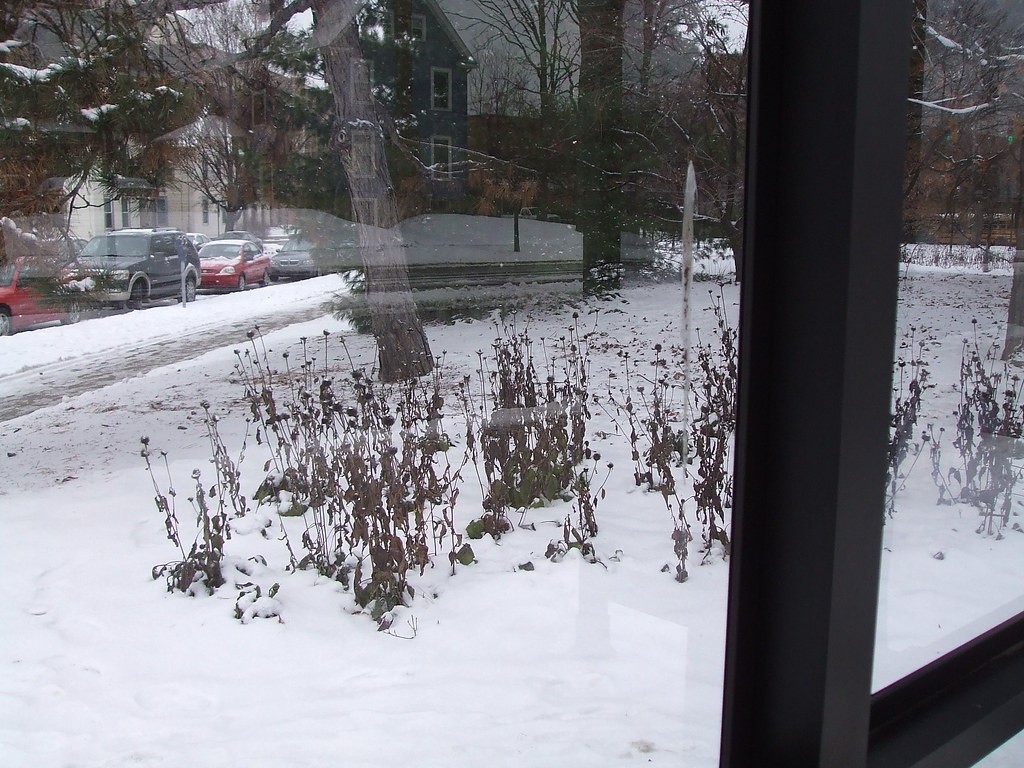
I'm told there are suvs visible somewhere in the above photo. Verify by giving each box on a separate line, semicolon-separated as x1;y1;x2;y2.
78;228;202;308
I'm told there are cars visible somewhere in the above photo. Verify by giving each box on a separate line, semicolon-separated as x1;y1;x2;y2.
40;238;91;256
196;240;273;293
0;255;86;337
186;232;211;250
211;232;263;253
270;235;326;281
